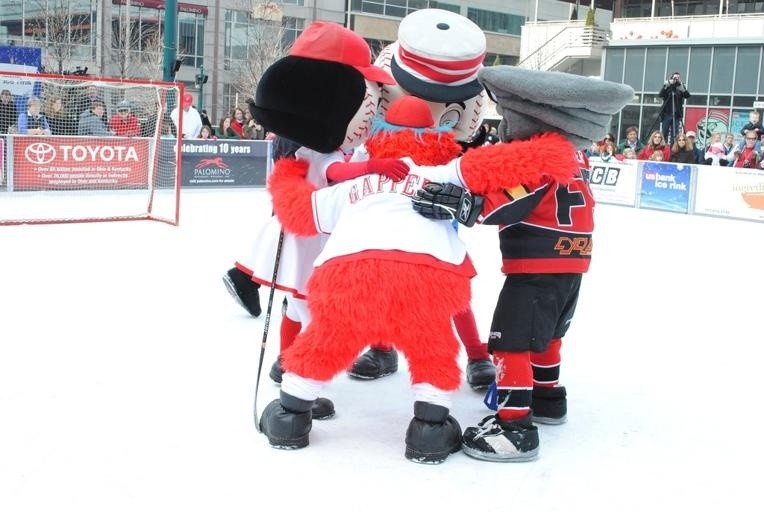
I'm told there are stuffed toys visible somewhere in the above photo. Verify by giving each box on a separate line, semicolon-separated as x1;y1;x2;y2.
410;61;636;463
220;21;410;388
259;92;580;466
346;7;507;391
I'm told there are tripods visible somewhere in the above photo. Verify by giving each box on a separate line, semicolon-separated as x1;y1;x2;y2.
642;88;686;146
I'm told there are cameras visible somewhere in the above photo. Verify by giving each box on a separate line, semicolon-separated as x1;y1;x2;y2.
744;161;751;168
674;80;681;88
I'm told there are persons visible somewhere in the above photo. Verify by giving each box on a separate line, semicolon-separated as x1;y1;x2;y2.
1;84;142;138
145;99;177;138
659;72;690;145
734;112;764;157
169;94;202;139
192;104;266;140
586;126;764;170
